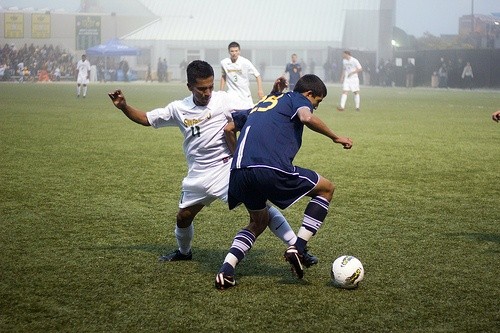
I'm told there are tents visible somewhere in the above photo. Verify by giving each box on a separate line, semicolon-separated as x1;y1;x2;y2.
85;39;142;81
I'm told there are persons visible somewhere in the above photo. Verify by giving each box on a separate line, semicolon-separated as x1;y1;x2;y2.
492;110;500;123
300;58;339;83
439;57;474;89
179;60;188;82
221;42;263;109
285;53;303;92
108;61;319;268
157;58;168;82
92;55;129;82
361;59;417;87
0;43;77;84
146;62;153;83
337;50;363;110
215;75;353;290
76;54;91;97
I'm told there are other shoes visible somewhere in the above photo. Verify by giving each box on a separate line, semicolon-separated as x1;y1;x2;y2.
215;272;239;290
158;249;193;262
299;246;318;267
284;245;304;278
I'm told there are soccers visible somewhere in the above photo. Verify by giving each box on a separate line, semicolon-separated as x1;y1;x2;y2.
330;255;365;290
